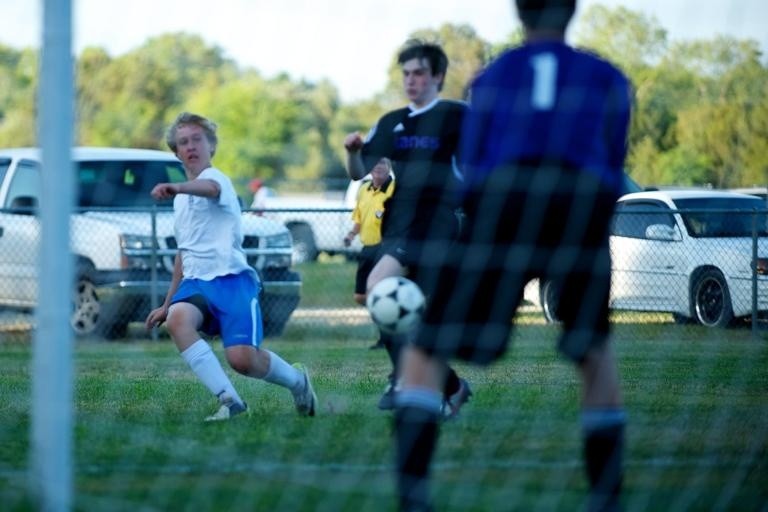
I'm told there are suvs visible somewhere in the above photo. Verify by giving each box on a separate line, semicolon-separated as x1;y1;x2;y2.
0;146;302;340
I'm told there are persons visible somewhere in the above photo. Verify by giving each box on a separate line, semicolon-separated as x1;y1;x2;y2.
396;0;642;512
344;44;498;427
344;156;399;304
145;113;321;423
249;178;278;213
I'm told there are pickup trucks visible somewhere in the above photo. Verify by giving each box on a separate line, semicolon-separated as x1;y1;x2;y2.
254;173;395;268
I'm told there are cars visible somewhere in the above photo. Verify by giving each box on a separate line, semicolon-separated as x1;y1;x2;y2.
722;188;768;201
523;191;768;329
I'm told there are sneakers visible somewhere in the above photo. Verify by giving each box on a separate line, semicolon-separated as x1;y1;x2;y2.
292;360;318;418
379;371;395;409
440;377;473;418
204;397;250;421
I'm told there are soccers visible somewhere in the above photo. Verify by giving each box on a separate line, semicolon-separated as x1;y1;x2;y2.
367;277;424;334
